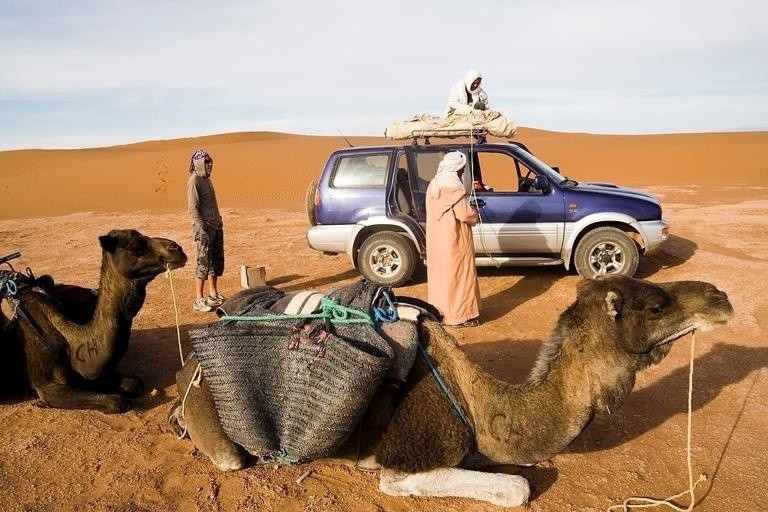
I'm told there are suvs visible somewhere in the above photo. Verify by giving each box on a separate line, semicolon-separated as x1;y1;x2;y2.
305;127;671;289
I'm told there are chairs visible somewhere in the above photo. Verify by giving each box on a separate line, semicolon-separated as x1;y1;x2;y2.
396;168;416;214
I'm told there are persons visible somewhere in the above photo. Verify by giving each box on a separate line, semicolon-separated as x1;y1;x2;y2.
187;149;225;313
445;68;491;117
424;150;482;328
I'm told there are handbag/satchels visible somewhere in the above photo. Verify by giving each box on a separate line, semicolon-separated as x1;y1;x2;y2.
188;314;395;461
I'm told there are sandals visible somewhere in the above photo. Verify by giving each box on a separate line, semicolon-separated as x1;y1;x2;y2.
452;320;480;327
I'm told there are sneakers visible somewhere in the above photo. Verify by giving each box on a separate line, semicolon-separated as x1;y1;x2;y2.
207;292;227;307
193;298;212;312
